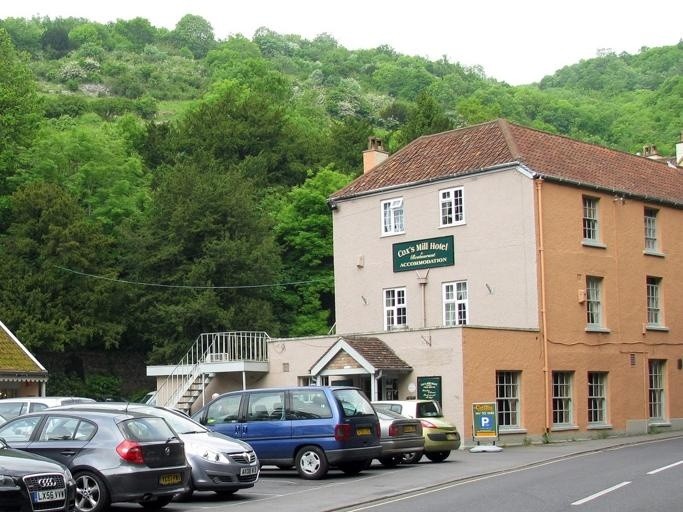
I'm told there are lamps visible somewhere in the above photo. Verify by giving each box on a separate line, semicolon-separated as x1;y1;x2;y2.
328;201;337;209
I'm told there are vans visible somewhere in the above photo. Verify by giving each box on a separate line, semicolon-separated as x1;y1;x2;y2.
190;386;384;480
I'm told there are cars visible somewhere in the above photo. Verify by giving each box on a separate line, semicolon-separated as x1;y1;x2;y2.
369;400;462;465
0;396;99;438
62;402;261;501
0;436;77;512
0;408;193;512
370;407;426;468
